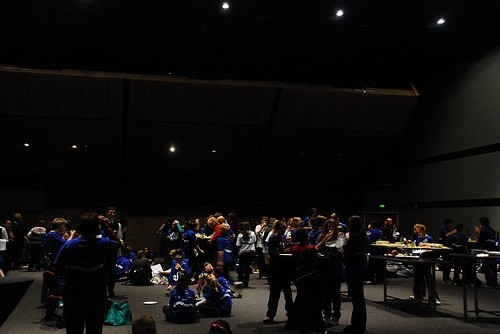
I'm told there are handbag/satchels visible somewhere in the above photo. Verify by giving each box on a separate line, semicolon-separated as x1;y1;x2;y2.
104;296;132;325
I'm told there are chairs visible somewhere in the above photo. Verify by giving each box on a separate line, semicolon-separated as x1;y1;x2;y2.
41;271;64;329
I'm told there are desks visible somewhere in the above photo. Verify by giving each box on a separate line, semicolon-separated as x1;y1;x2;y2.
365;242;500;323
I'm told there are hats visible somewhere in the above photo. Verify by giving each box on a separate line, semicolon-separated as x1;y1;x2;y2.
173;275;190;285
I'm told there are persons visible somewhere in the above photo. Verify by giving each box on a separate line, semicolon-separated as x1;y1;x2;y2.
409;224;441;305
163;275;198;324
343;215;369;332
54;212;122;334
0;208;499;334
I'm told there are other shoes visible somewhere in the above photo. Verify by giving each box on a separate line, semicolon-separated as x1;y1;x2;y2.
323;318;330;327
263;317;274;323
441;279;495;287
43;317;55;322
343;325;355;334
327;317;341;326
436;298;441;304
410;296;414;299
